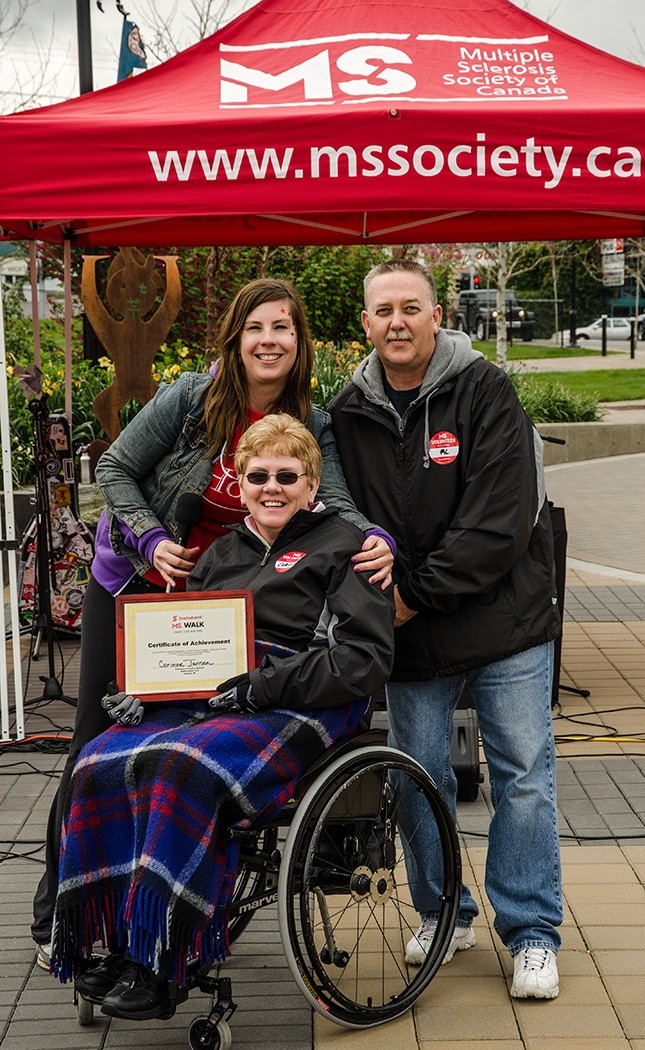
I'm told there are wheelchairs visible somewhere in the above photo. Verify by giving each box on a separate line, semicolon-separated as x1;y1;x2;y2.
76;724;462;1050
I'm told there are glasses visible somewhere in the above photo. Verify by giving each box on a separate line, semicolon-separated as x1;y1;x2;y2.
243;472;308;485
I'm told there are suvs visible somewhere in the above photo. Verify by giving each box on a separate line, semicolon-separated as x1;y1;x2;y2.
453;292;535;342
553;318;632;339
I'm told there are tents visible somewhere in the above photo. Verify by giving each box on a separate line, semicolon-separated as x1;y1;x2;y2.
0;0;645;744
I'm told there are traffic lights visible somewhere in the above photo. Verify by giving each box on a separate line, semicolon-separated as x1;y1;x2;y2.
455;277;461;294
473;274;481;290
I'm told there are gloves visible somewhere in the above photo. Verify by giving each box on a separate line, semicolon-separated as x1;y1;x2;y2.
207;673;262;714
101;681;144;726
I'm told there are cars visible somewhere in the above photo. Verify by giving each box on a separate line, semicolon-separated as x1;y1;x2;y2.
634;314;645;340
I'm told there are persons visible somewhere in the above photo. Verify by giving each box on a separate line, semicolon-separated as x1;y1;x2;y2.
322;259;565;1002
49;414;397;1028
32;279;396;972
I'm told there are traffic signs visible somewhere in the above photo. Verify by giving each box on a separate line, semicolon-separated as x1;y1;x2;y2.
601;239;625;253
601;270;624;287
601;254;624;269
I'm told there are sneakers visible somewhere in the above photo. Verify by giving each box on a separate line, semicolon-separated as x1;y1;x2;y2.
510;947;559;998
100;965;189;1020
74;951;133;1001
35;941;52;971
404;919;477;965
